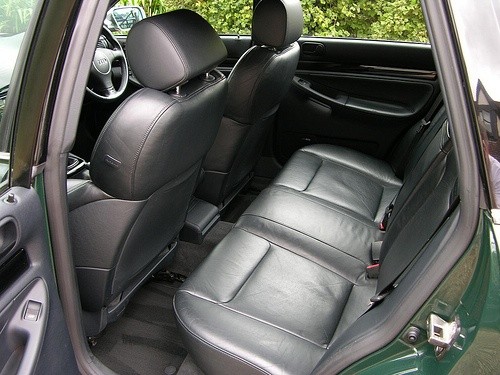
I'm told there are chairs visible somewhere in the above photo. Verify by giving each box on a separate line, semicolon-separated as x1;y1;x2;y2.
67;2;232;310
190;1;305;211
171;106;459;375
